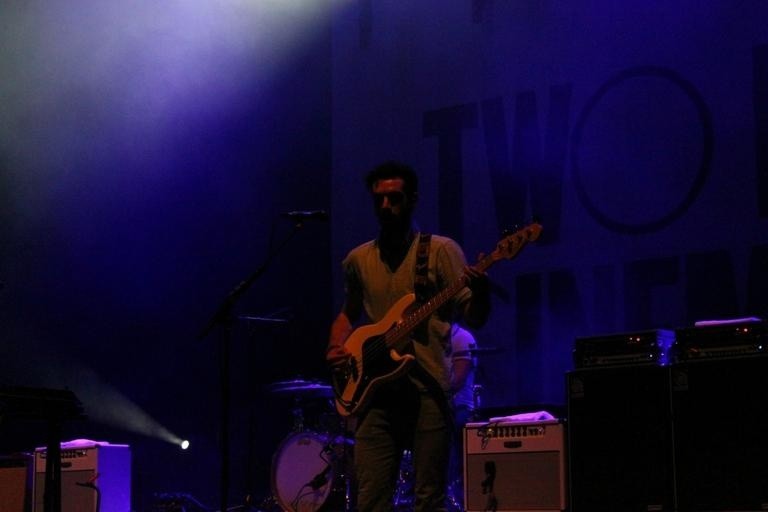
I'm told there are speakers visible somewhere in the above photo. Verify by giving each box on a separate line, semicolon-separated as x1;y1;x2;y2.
462;418;567;511
30;444;133;512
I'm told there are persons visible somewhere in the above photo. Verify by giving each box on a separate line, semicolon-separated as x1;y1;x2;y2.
322;162;492;512
440;318;480;455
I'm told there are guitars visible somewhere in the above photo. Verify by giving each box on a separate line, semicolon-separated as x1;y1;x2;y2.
334;223;543;417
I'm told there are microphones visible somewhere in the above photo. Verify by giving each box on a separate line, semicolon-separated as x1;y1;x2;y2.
285;210;330;222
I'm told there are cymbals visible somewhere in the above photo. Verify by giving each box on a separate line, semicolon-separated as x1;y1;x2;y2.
452;347;497;359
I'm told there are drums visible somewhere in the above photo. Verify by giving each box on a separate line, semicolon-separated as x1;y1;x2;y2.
273;432;356;511
261;379;356;438
